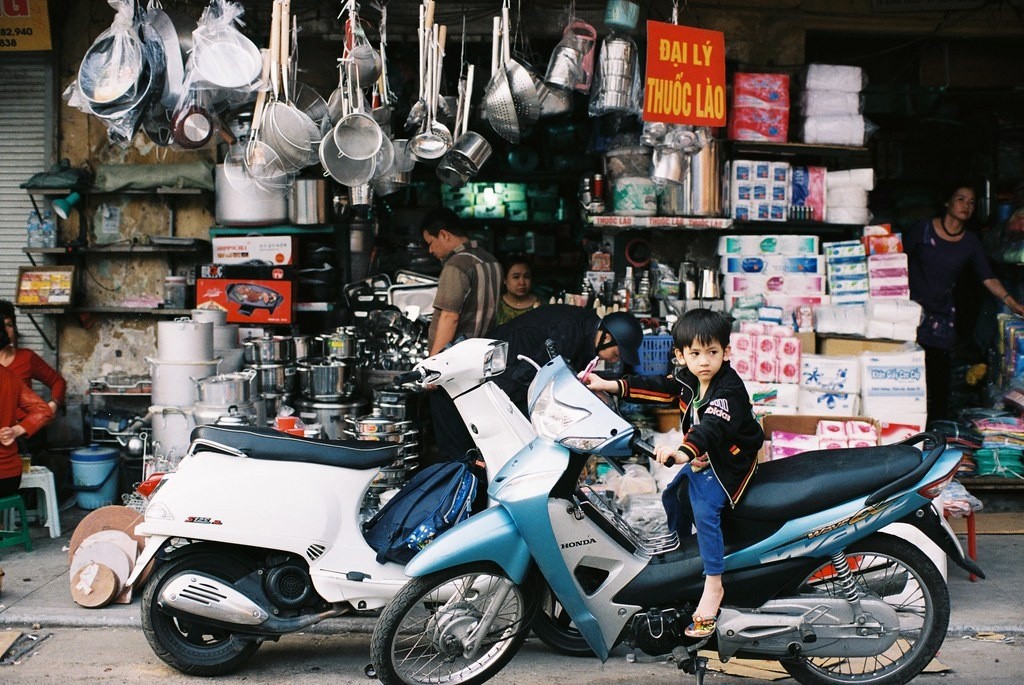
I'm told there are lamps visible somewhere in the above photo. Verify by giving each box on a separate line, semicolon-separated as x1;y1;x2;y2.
52;192;90;246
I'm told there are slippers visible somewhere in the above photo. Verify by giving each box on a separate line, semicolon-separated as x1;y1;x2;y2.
683;608;717;638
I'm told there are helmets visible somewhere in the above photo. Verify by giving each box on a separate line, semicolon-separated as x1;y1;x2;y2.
603;312;643;365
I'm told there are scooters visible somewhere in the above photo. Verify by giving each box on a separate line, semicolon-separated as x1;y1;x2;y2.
120;336;644;676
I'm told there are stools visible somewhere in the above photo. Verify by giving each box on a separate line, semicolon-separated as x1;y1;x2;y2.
5;465;60;538
0;496;32;551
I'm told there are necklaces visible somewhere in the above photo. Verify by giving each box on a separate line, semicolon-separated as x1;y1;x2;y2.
941;216;963;236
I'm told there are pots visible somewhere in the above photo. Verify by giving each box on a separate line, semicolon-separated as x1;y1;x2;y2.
53;0;719;613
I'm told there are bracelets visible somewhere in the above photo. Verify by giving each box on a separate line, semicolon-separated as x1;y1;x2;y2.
1001;294;1010;301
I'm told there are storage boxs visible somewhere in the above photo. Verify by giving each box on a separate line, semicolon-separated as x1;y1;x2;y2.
16;265;82;308
196;235;294;325
637;62;929;463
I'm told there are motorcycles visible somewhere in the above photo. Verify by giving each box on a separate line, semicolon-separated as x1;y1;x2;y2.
370;340;986;685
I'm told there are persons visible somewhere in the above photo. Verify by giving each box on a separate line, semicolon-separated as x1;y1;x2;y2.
492;255;545;329
893;181;1024;422
419;208;501;460
577;308;764;638
0;300;67;497
482;304;643;422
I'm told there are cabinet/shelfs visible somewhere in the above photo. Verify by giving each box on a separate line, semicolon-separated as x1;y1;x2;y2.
89;373;158;476
19;186;210;353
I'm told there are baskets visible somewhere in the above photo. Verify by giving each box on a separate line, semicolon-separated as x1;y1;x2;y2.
634;335;675;375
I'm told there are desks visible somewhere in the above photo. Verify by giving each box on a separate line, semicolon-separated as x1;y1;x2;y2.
939;483;985;581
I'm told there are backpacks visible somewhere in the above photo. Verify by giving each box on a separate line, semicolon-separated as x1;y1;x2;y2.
363;450;478;569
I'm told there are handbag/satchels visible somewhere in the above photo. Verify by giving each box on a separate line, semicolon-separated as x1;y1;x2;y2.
917;314;957;350
